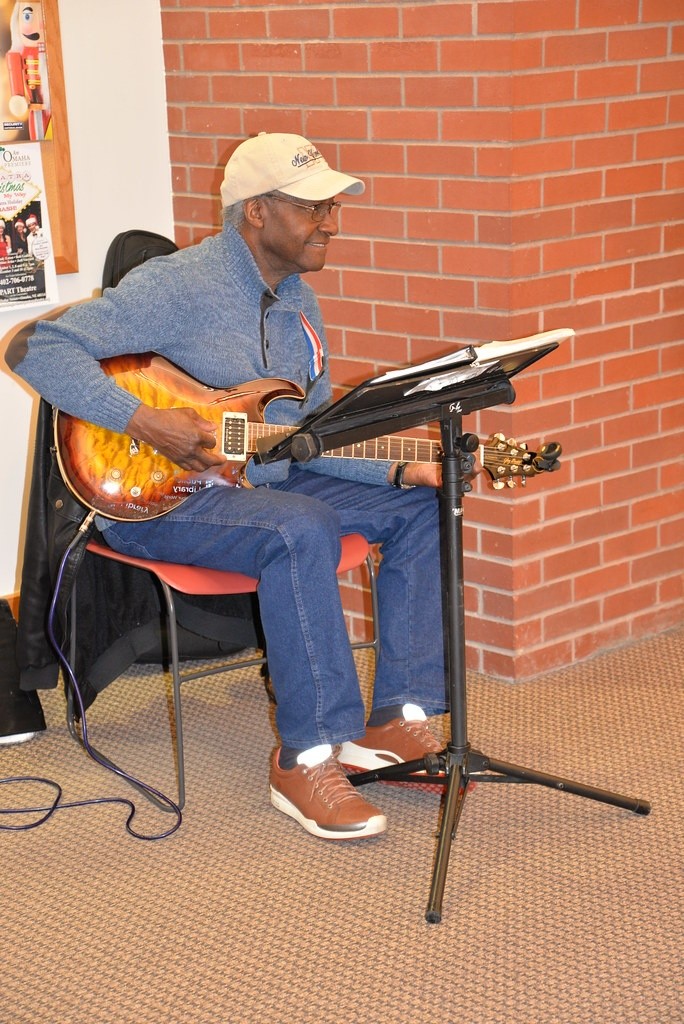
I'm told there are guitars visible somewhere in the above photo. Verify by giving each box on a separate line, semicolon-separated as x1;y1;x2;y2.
52;352;562;521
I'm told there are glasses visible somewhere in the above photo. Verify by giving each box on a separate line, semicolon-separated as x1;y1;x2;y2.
265;195;342;222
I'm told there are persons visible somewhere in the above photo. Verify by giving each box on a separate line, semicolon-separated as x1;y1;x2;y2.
4;132;481;839
12;218;28;254
0;220;12;267
26;214;45;293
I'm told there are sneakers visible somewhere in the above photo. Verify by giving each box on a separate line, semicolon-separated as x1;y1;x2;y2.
338;703;476;795
268;745;386;841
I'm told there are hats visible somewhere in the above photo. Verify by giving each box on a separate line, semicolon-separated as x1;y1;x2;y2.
25;214;38;230
219;132;366;207
15;219;27;232
0;220;5;228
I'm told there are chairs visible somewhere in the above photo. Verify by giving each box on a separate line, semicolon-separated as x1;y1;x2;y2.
16;231;384;808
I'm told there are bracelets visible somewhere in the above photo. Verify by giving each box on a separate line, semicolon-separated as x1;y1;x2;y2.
394;462;416;490
